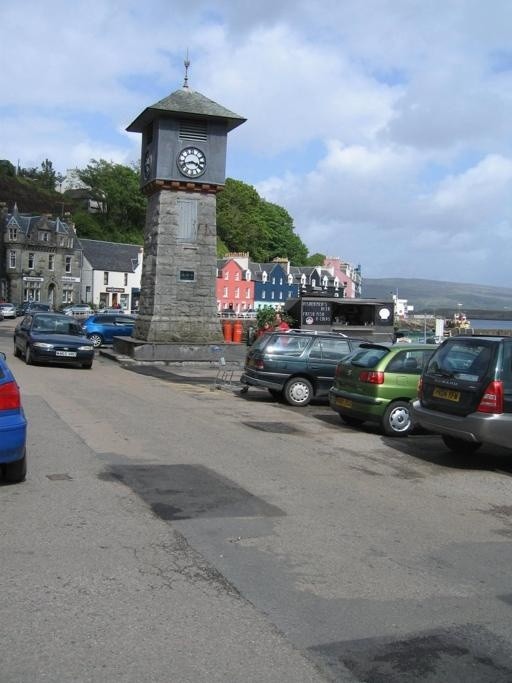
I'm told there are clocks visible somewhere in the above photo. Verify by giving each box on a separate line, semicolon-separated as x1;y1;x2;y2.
176;144;208;178
143;149;152;179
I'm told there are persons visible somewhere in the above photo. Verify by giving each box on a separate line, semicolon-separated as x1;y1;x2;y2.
273;312;290;330
259;321;273;338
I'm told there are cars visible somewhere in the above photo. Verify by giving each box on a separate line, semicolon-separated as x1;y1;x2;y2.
0;300;140;369
0;349;30;483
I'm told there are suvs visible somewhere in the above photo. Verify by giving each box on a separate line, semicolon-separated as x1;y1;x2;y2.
240;327;512;456
